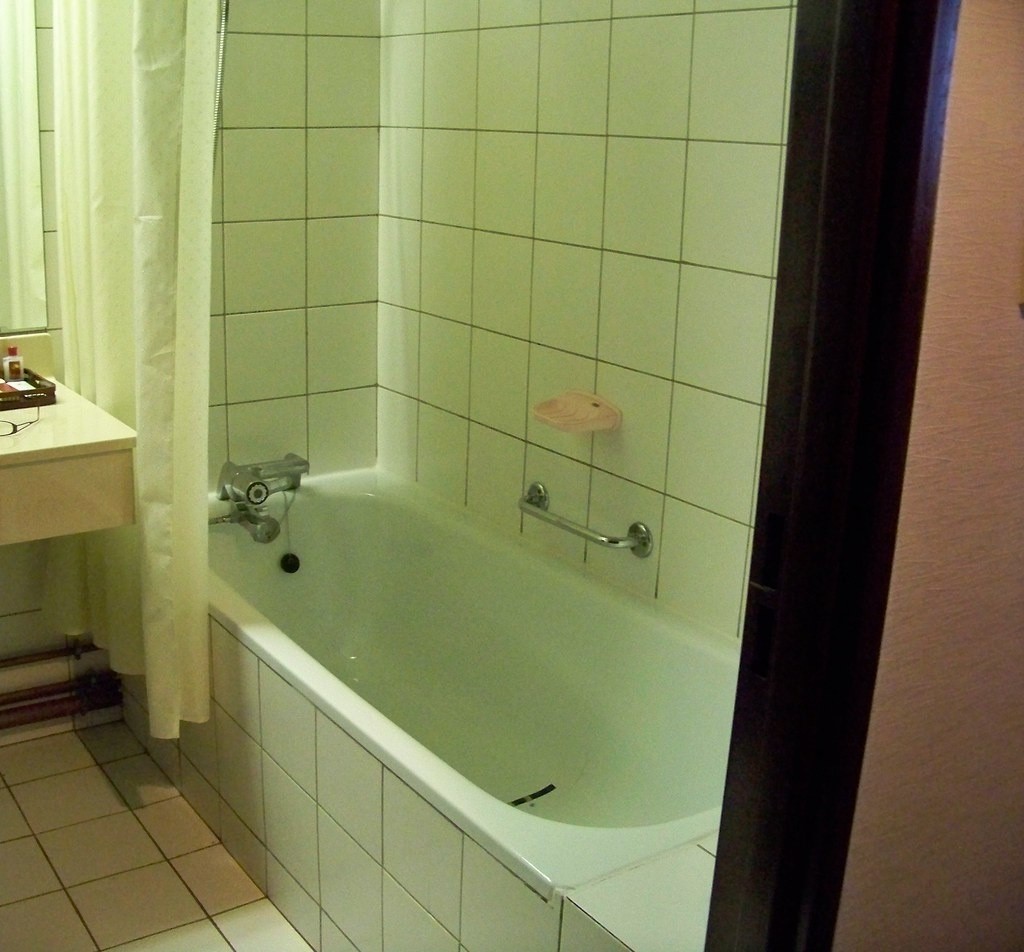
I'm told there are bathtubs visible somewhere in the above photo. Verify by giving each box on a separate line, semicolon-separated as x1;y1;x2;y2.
122;467;742;951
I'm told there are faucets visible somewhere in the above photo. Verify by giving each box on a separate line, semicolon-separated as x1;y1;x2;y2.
227;501;282;543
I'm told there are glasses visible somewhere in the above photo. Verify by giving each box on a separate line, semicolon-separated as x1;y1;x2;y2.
0;405;39;436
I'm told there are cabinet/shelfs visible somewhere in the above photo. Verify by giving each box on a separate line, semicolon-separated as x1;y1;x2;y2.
0;375;141;546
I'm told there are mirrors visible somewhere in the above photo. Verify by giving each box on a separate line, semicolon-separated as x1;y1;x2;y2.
0;0;49;339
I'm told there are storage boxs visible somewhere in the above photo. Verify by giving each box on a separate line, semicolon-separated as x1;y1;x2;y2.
0;366;56;413
